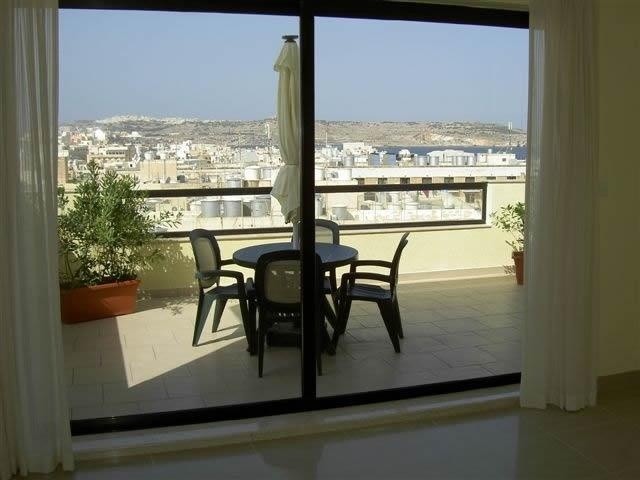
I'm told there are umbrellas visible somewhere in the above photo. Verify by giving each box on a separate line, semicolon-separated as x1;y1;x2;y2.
268;29;301;252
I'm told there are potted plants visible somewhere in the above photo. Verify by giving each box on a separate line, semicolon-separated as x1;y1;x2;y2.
489;202;526;285
59;157;170;326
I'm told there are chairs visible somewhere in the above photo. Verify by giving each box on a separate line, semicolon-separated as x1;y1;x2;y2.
189;217;410;376
